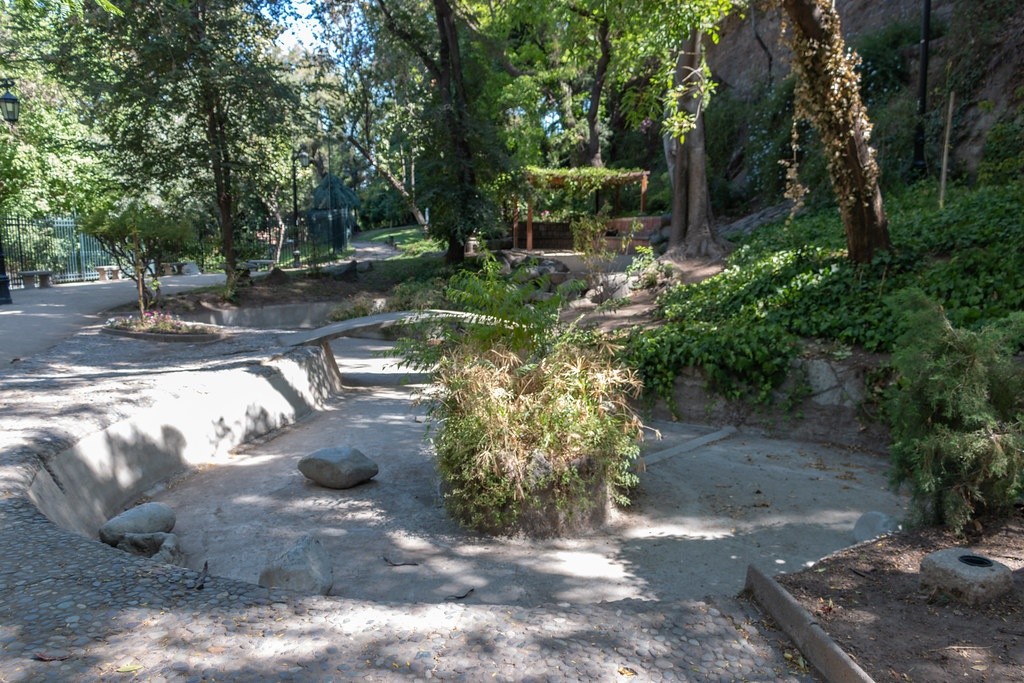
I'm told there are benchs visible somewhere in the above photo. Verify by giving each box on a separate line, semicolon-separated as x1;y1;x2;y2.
94;266;124;281
590;236;650;253
18;271;54;289
249;260;275;271
161;262;187;275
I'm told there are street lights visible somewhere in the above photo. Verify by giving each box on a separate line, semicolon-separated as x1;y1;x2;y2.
292;152;309;268
0;81;21;301
